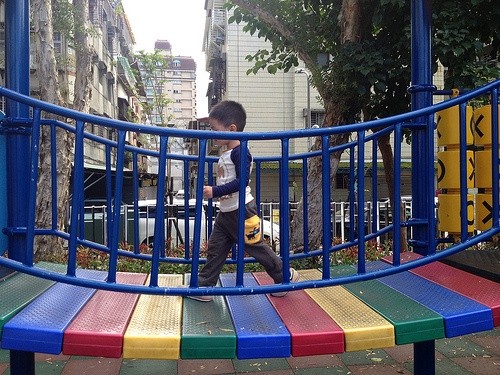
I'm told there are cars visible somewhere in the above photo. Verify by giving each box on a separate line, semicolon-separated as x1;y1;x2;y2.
175;190;191;199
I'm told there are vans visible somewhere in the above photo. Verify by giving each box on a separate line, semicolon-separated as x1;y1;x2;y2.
132;198;279;253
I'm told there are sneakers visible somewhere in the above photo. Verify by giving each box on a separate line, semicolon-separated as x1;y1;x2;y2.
181;285;213;302
270;268;298;297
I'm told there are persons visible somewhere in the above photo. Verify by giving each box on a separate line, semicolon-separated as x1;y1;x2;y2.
179;101;298;302
